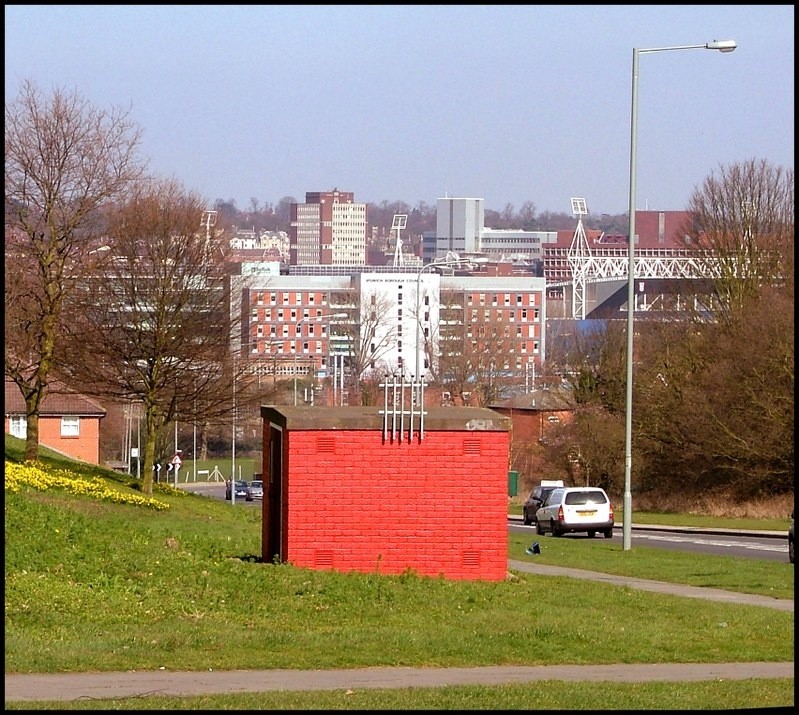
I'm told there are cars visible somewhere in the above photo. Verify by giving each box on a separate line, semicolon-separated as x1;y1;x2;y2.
246;480;264;502
225;478;251;500
522;479;564;526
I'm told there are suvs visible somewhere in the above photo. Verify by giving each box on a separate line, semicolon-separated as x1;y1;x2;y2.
534;487;615;539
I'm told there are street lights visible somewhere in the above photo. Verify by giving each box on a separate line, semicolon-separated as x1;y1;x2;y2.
174;366;201;489
414;257;491;410
230;339;287;505
621;39;739;554
